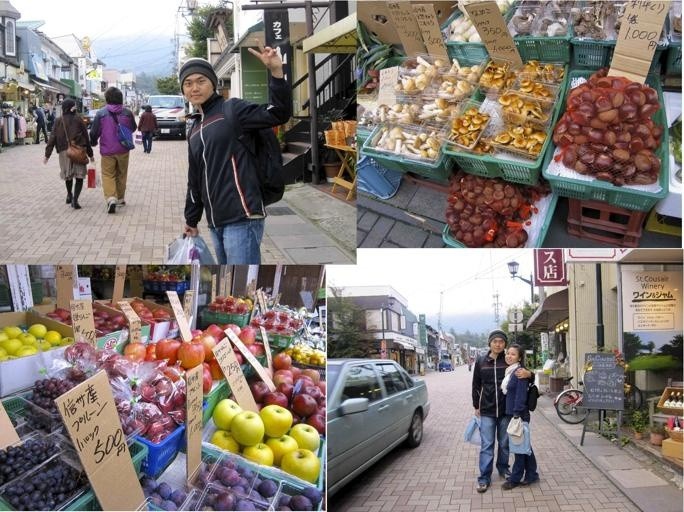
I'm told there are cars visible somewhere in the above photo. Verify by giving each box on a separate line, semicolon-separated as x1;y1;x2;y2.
83;108;100;126
327;356;428;499
439;360;453;372
138;110;144;117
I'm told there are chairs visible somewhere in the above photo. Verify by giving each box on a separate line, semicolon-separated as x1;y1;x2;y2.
644;378;683;430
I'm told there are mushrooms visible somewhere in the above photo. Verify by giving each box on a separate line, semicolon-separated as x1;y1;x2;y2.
377;56;662;248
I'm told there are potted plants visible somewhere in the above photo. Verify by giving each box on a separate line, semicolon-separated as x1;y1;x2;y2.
631;421;645;441
648;424;666;446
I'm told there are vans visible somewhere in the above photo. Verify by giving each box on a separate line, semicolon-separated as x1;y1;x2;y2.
140;94;188;139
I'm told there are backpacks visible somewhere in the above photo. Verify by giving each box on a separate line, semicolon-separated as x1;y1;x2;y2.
254;129;286;207
527;383;539;411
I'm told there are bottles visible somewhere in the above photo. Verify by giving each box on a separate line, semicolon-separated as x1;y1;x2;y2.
663;391;683;432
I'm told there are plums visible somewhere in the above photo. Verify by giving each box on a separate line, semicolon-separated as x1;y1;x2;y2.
139;458;322;511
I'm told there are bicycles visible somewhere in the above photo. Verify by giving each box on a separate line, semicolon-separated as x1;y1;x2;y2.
553;376;643;425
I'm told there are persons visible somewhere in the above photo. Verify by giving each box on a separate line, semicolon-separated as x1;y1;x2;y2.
177;37;294;264
500;340;539;491
51;93;65;130
31;104;48;145
89;86;138;214
42;97;95;210
470;330;536;496
138;106;158;153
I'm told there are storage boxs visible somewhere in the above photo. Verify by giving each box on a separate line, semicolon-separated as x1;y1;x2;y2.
656;386;683;417
661;437;684;460
0;291;181;398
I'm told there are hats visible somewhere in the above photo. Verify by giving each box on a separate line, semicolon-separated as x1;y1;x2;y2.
488;330;508;347
178;57;217;94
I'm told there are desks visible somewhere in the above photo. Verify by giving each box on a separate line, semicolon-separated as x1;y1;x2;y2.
323;142;358;201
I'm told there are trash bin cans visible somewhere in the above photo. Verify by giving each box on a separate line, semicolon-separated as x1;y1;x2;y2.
549;376;566;395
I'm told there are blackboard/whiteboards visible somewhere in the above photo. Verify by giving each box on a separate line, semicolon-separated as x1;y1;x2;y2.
584;353;626;410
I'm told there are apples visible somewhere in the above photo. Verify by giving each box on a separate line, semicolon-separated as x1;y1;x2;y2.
0;297;326;484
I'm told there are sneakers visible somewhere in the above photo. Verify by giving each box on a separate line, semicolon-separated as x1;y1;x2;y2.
106;196;116;214
115;198;125;207
501;479;519;490
520;474;540;486
475;481;491;493
498;470;513;481
66;194;73;204
70;200;81;209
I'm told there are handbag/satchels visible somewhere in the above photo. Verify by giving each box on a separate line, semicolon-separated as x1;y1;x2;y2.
464;416;478;441
66;140;90;164
506;413;526;438
165;232;217;265
87;166;96;189
470;424;482;445
506;420;533;456
118;122;135;150
